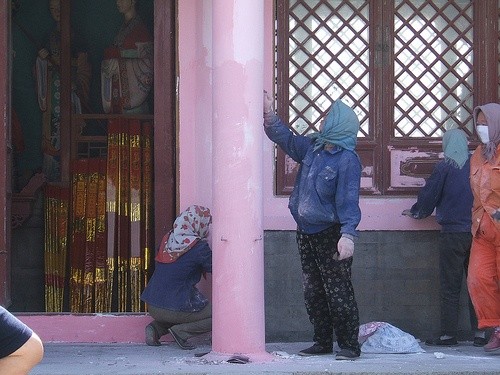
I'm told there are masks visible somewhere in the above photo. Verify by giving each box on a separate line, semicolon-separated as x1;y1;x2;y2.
476;125;490;143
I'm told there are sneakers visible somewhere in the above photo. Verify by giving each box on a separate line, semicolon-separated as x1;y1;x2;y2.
483;330;500;351
298;344;332;356
335;350;359;360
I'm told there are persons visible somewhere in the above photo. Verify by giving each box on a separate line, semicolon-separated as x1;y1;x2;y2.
264;89;362;359
141;205;212;349
101;0;152;115
402;129;492;346
466;104;500;351
36;0;92;181
0;306;43;375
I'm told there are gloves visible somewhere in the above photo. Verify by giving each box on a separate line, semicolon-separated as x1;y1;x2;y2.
264;90;272;113
337;236;354;261
402;209;412;216
490;210;500;221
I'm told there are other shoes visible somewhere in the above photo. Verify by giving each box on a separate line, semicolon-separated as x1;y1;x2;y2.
168;327;197;349
426;336;457;346
473;337;488;347
145;324;161;346
